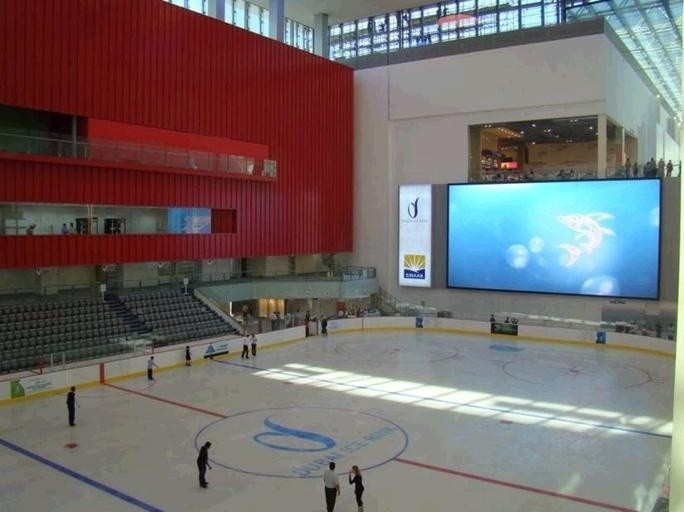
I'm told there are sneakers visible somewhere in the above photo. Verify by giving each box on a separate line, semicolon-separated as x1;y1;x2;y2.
200;481;210;489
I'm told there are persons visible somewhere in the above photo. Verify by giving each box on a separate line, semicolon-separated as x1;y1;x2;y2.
489;313;496;322
183;346;192;366
320;316;327;337
623;156;674;179
250;333;257;355
348;465;364;512
503;317;509;323
239;302;310;331
195;440;212;488
64;386;79;427
61;222;87;236
510;317;518;324
322;461;341;512
613;317;674;340
240;334;249;359
146;355;158;379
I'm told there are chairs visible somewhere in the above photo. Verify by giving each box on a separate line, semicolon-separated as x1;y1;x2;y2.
0;287;238;375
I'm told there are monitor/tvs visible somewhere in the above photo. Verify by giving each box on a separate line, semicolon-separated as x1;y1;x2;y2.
446;177;662;301
